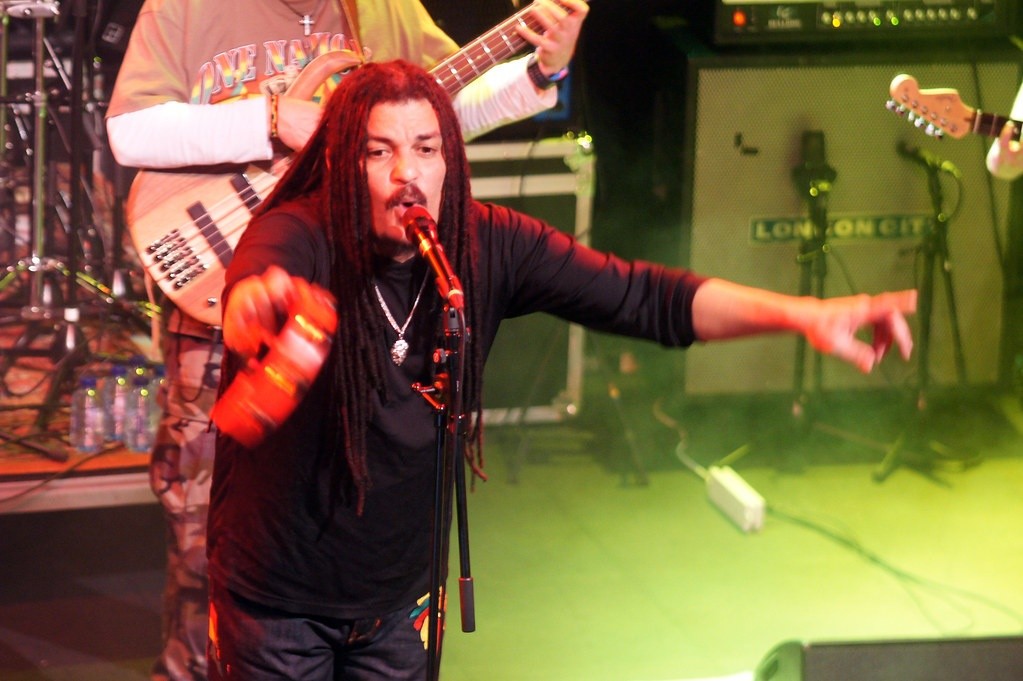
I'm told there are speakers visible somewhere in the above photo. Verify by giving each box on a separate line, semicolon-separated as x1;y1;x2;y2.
640;12;1023;411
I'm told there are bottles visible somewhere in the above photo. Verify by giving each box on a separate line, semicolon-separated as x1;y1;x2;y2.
126;355;149;380
69;373;105;451
149;365;167;436
125;377;157;451
101;363;134;440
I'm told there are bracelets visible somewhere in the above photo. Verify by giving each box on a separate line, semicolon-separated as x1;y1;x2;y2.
270;93;278;142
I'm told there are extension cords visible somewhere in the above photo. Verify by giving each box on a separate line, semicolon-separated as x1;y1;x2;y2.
705;464;767;533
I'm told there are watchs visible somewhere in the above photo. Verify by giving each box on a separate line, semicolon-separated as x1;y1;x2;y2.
527;56;569;91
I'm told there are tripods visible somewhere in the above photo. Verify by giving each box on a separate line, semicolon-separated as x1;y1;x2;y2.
704;184;931;470
850;169;1023;483
0;0;163;338
0;0;163;429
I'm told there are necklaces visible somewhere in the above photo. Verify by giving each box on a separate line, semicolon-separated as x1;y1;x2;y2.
359;257;433;369
282;0;321;37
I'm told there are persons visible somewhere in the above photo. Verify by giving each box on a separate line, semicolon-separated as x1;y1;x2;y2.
102;1;592;681
985;79;1023;184
216;61;916;677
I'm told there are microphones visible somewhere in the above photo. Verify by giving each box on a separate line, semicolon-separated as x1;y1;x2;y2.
796;129;832;202
895;138;960;178
401;206;464;309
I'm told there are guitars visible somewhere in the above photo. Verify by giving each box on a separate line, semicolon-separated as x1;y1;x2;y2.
127;0;590;325
887;74;1023;143
